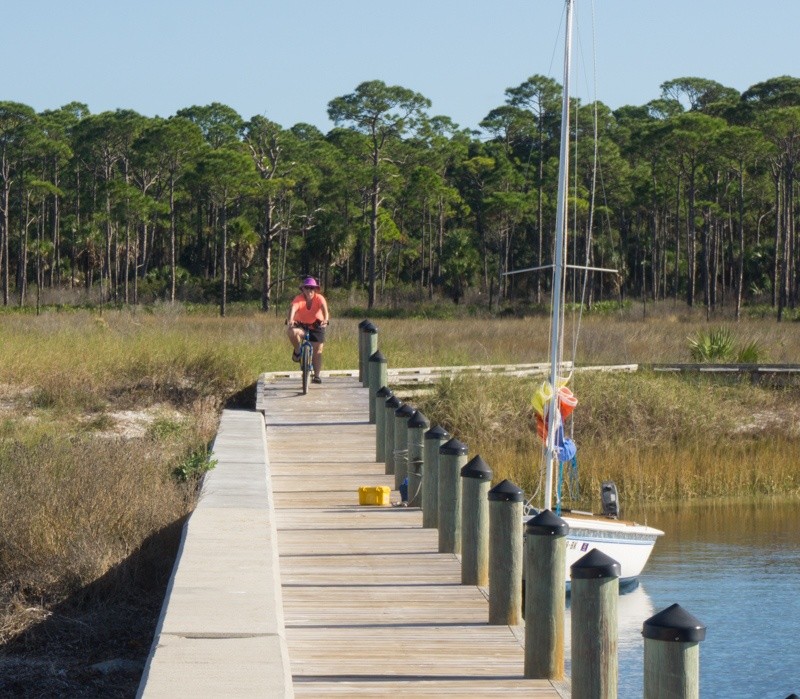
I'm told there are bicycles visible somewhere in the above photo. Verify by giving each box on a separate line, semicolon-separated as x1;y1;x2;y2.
284;319;330;395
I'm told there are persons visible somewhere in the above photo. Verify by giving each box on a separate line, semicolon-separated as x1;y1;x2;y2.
287;278;329;384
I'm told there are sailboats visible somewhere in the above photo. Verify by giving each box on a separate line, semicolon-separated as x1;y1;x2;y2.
501;0;668;595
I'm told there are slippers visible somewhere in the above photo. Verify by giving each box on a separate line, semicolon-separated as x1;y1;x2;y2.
314;376;321;383
292;349;301;362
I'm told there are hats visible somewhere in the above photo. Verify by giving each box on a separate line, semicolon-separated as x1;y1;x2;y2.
299;278;320;291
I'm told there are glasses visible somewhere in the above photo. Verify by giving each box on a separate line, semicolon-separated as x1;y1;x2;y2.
304;287;315;290
